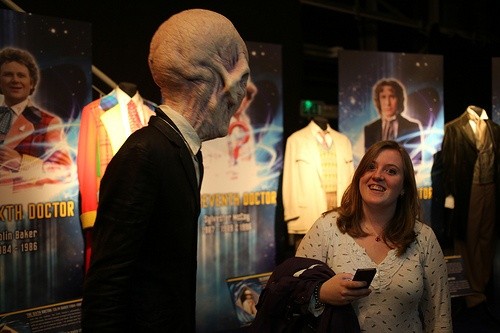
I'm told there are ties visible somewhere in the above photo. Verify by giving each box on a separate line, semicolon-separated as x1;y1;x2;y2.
472;118;492;185
320;130;328;148
127;100;143;132
383;119;397;141
0;107;12;134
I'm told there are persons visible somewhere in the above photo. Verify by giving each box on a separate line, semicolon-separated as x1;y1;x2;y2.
295;141;454;333
81;9;251;333
363;79;422;177
439;105;500;311
0;47;73;184
77;82;158;280
283;118;354;254
244;289;257;314
227;80;262;164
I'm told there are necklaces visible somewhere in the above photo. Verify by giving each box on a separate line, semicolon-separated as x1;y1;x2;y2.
362;221;384;241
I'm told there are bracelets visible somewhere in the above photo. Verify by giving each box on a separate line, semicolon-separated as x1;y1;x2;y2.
315;283;327;308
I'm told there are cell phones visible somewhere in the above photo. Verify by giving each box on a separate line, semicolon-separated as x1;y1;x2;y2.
352;268;377;290
